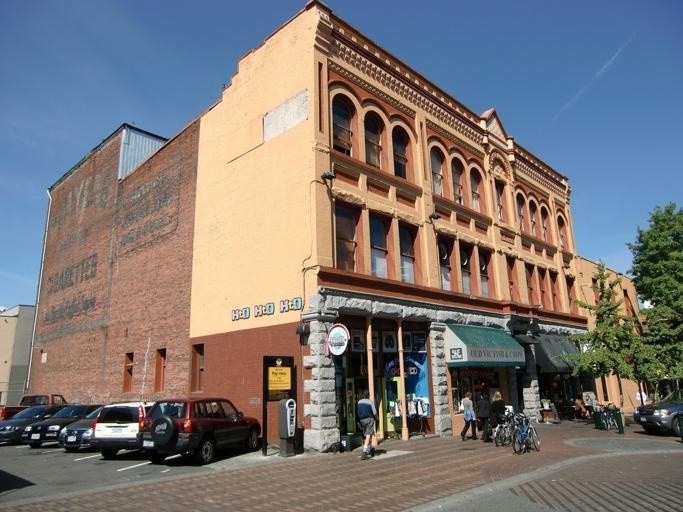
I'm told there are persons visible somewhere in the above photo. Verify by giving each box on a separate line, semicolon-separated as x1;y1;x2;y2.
459;392;477;441
475;393;495;441
491;391;504;414
356;388;378;459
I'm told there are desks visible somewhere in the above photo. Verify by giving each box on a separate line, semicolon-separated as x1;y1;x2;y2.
538;408;554;424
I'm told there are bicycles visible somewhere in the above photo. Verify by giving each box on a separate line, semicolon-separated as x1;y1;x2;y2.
596;402;618;432
512;415;541;455
499;410;523;446
494;407;524;447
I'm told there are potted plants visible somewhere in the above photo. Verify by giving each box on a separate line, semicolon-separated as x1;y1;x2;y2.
540;398;551;408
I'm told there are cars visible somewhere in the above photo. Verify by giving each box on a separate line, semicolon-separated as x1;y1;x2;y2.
20;405;105;448
58;406;104;453
0;404;72;445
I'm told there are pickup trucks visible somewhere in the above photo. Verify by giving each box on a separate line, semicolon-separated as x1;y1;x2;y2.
0;393;76;421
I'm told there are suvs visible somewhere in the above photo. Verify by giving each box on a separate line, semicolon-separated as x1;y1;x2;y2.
136;397;260;465
633;389;682;435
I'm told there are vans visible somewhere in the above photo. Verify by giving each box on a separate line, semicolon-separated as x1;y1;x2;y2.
89;401;183;460
